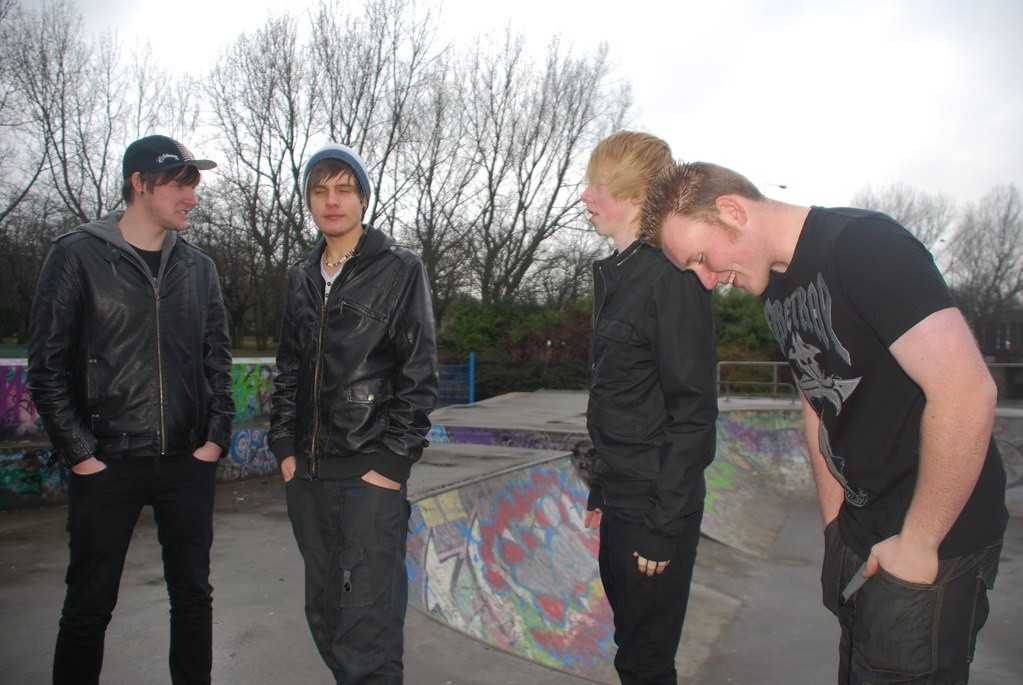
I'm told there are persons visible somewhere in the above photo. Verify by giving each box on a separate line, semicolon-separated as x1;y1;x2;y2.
633;159;1011;685
28;134;237;685
264;142;436;683
568;129;717;685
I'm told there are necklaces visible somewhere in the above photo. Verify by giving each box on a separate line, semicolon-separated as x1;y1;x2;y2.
322;245;357;268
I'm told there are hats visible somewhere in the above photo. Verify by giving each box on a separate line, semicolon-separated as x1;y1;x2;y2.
122;134;218;180
302;144;372;215
